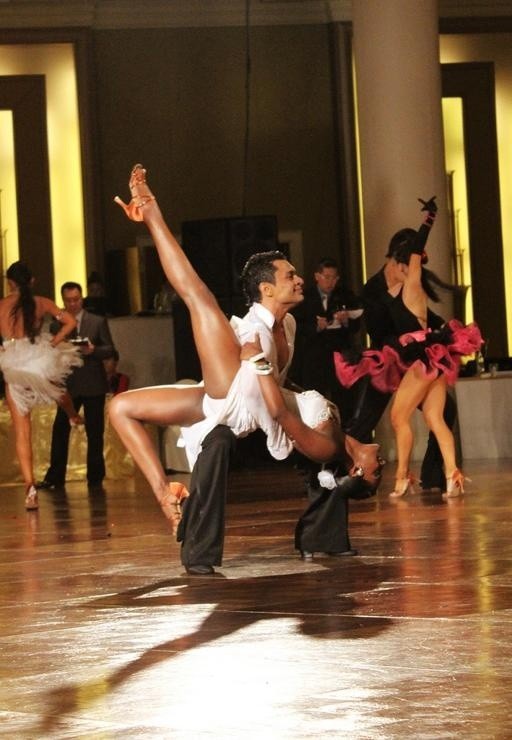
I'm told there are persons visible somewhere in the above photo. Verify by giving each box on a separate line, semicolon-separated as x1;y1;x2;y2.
108;162;388;544
177;247;360;576
0;259;89;510
33;281;119;493
325;193;483;502
282;252;360;475
83;272;114;318
317;227;463;492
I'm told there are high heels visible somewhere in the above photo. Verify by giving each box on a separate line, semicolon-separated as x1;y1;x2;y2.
388;476;416;499
440;467;472;500
159;480;191;543
23;482;40;510
69;413;87;434
114;163;156;222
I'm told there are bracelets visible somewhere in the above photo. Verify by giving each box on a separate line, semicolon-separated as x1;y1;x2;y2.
422;222;432;227
250;352;274;376
425;214;434;220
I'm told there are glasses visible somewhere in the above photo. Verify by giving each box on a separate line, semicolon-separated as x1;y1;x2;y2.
320;275;340;281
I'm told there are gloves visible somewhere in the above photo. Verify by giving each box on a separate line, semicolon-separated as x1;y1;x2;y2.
411;195;438;254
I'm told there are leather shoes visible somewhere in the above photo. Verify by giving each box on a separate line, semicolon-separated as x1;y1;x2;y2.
302;548;358;558
187;564;215;574
